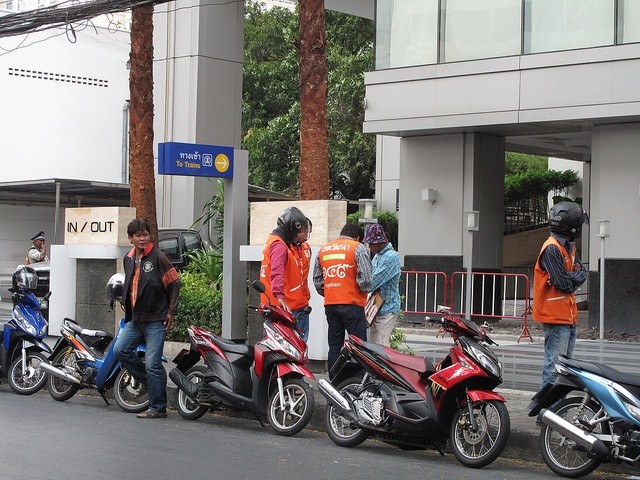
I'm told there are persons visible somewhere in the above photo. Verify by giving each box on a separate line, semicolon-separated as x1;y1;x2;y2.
364;223;401;349
114;218;181;417
260;207;307;340
533;203;588;427
25;231;50;265
313;224;372;380
292;219;311;343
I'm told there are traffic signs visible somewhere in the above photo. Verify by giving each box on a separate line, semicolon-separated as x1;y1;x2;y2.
158;142;233;178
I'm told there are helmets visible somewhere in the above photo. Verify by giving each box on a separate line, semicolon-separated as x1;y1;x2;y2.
12;266;38;291
549;202;589;239
277;207;307;243
107;272;127;300
295;217;312;243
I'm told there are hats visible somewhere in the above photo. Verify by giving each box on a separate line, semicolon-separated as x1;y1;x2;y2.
30;231;45;243
364;224;388;244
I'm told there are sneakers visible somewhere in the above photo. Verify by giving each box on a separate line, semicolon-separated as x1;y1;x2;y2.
535;417;544;426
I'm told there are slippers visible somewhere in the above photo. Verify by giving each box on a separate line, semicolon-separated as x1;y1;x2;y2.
136;408;167;418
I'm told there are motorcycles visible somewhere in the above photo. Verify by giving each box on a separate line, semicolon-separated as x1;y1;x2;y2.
39;296;167;413
0;288;51;395
169;280;314;436
526;354;640;478
317;305;510;469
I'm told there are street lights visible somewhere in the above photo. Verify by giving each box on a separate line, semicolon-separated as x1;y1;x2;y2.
358;198;378;258
595;219;611;341
465;211;479;322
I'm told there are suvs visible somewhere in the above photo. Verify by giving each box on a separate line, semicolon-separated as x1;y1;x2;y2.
26;228;208;322
